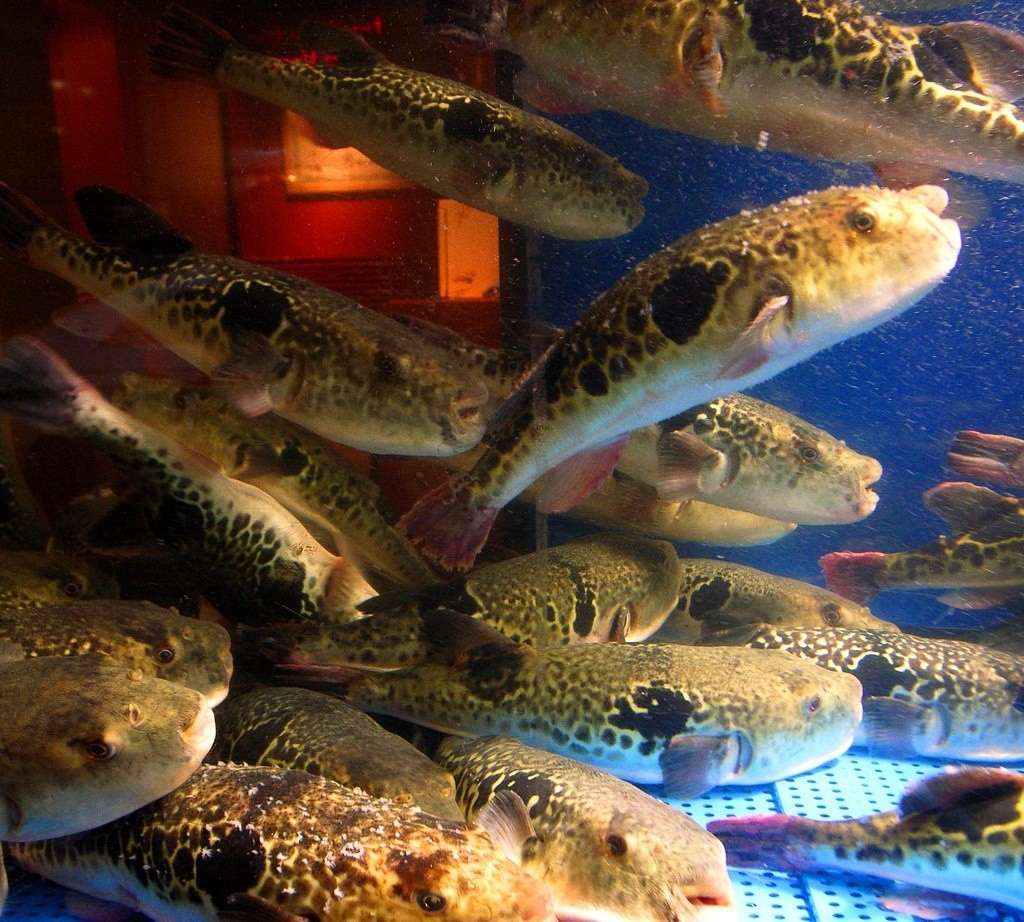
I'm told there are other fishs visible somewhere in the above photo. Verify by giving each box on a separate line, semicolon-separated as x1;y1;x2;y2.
0;0;1024;922
